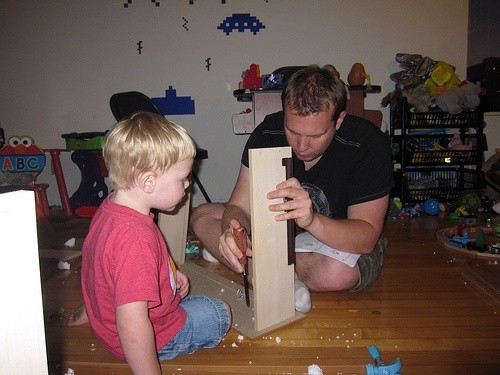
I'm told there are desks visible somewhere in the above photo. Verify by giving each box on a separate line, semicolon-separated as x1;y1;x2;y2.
232;85;382;134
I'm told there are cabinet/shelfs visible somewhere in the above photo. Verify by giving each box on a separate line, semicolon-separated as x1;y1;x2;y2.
390;96;488;212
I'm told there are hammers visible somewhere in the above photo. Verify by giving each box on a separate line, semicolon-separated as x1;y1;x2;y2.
365;345;401;375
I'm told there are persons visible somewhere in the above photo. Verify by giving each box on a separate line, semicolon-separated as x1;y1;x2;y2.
81;110;231;375
190;67;393;313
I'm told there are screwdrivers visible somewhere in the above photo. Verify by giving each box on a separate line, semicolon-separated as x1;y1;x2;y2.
233;225;250;307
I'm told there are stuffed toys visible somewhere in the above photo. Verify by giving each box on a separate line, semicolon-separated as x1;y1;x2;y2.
381;54;480;124
